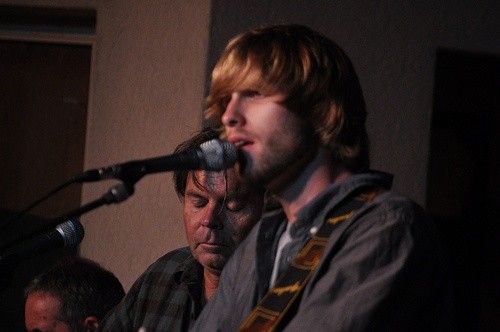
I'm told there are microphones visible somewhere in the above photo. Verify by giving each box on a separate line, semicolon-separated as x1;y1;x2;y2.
1;219;84;267
80;140;237;184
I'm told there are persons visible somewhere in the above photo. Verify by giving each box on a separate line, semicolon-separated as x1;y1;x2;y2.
23;258;126;332
190;24;440;331
93;125;264;331
